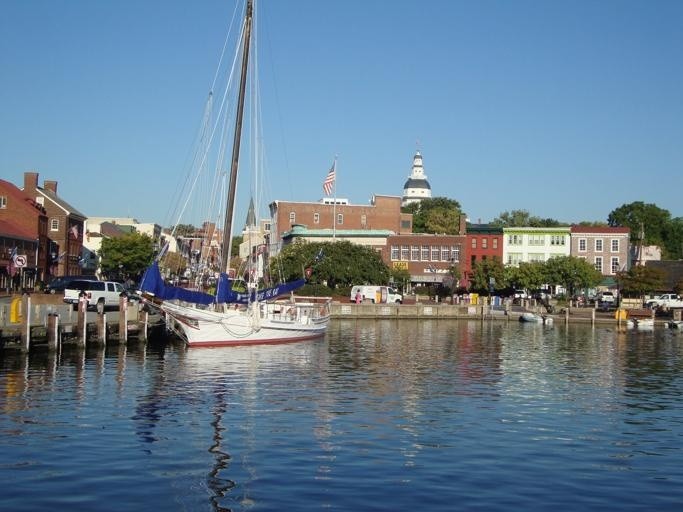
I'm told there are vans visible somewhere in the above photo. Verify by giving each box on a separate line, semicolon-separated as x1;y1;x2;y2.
350;285;402;305
43;275;131;312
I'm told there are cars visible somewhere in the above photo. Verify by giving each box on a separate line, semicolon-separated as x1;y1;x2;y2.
512;289;528;300
592;292;614;302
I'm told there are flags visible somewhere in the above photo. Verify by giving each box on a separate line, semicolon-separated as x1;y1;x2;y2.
70;223;79;239
9;247;17;261
322;161;335;196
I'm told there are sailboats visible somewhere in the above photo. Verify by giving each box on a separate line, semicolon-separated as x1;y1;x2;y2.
134;0;333;348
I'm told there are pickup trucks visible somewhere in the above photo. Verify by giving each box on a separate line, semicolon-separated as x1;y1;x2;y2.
646;294;683;310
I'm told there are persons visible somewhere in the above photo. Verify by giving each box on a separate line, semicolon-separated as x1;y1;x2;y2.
354;289;360;304
353;319;360;344
